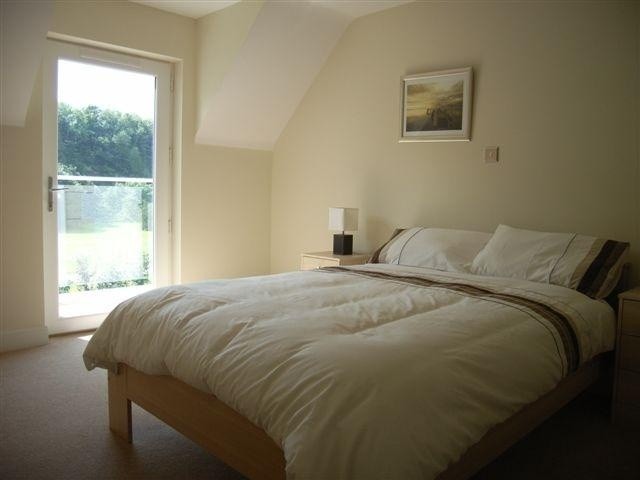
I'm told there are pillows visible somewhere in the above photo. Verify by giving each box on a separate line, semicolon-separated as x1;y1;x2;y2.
469;224;629;301
372;227;494;273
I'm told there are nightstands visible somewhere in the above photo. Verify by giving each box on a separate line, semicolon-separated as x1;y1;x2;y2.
299;250;370;270
609;287;640;432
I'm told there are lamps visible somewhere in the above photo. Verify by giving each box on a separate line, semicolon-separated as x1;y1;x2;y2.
328;206;359;256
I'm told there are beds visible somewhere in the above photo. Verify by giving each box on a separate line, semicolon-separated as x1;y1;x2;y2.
83;263;629;480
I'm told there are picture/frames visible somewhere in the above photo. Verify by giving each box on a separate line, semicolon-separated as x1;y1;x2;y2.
396;66;472;144
